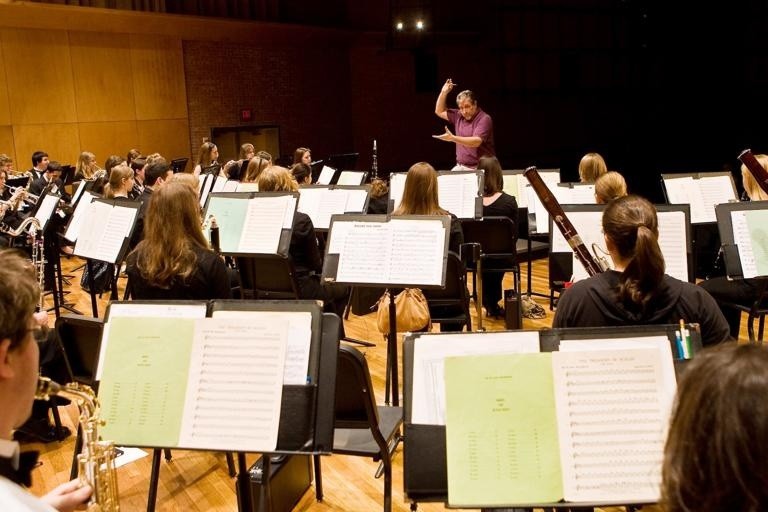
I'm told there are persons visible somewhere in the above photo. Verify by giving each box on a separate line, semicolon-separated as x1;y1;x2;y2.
651;343;766;512
366;177;389;214
72;150;105;182
27;147;77;190
697;149;767;346
428;78;498;172
0;152;34;195
0;170;39;243
593;171;627;205
105;148;180;199
193;140;317;194
1;249;95;512
550;196;739;345
390;162;471;334
28;162;74;208
125;182;236;298
577;151;609;187
472;154;519;318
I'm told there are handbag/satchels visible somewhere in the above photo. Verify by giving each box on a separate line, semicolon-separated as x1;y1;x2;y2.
370;288;430;333
520;294;546;319
80;259;113;294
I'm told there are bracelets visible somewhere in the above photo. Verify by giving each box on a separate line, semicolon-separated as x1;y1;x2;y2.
440;92;446;96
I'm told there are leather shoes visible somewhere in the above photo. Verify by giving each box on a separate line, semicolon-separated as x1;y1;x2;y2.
13;424;70;443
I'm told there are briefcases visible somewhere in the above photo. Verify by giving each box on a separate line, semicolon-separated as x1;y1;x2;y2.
234;453;313;511
503;290;520;329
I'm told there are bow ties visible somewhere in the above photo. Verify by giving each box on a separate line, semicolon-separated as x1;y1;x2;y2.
35;170;46;174
0;451;38;487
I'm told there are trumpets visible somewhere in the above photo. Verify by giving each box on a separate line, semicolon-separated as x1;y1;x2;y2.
4;184;40;204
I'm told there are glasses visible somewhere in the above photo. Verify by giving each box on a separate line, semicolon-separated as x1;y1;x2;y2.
26;324;49;343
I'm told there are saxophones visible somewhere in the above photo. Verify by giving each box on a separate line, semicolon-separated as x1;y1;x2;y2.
36;376;124;512
0;218;45;313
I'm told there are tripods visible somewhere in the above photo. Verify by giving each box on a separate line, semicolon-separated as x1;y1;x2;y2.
374;289;404;478
55;254;76;286
44;255;72;302
517;237;559;299
46;212;84;318
